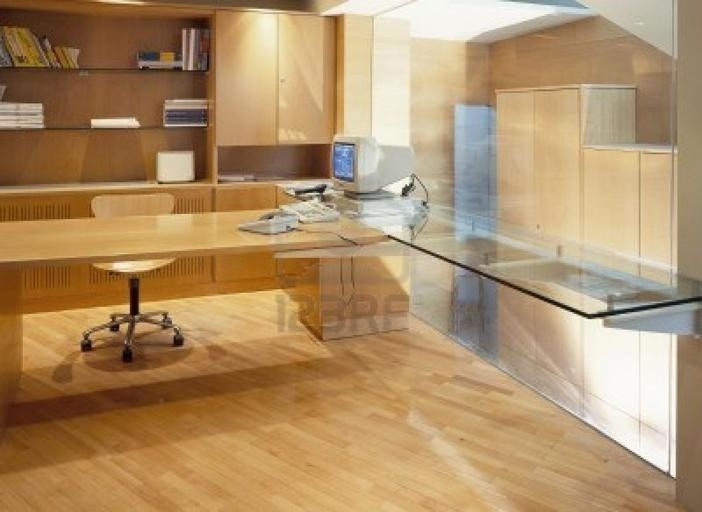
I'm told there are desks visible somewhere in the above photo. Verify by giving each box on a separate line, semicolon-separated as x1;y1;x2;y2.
1;209;407;435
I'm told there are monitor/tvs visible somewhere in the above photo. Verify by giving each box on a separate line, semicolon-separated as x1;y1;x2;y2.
330;134;414;199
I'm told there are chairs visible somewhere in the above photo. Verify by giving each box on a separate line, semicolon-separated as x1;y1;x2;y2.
77;190;181;367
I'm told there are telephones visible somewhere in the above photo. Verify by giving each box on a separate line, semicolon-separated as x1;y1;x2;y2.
239;212;299;234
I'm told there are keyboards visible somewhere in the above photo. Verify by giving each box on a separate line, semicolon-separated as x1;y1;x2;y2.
280;200;340;222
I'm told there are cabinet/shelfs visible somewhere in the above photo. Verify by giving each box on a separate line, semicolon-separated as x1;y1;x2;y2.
212;10;337;145
496;85;635;424
216;186;315;279
581;144;676;479
1;7;216;182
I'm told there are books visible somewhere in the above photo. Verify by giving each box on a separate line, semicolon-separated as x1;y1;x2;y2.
163;98;209;128
136;28;208;71
0;26;80;69
91;117;142;129
0;84;45;129
